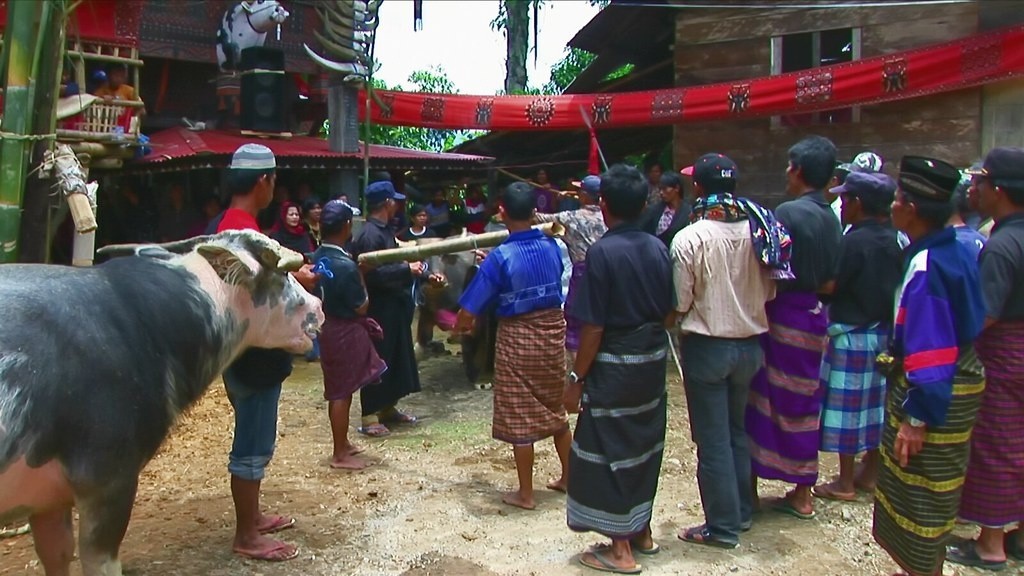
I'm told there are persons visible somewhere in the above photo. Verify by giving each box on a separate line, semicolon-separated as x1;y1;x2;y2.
47;53;1024;576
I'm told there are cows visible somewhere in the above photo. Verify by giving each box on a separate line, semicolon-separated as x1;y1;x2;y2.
216;0;287;71
0;228;326;575
395;227;493;390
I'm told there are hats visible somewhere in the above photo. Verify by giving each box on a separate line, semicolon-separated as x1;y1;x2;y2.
228;142;1024;228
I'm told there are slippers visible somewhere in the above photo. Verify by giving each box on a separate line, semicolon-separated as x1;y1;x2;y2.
579;549;642;573
943;538;1008;570
378;410;417;427
809;484;856;501
833;472;876;493
1002;528;1024;560
776;495;817;520
254;514;295;534
357;424;391;436
678;523;740;549
233;537;300;562
629;536;660;554
